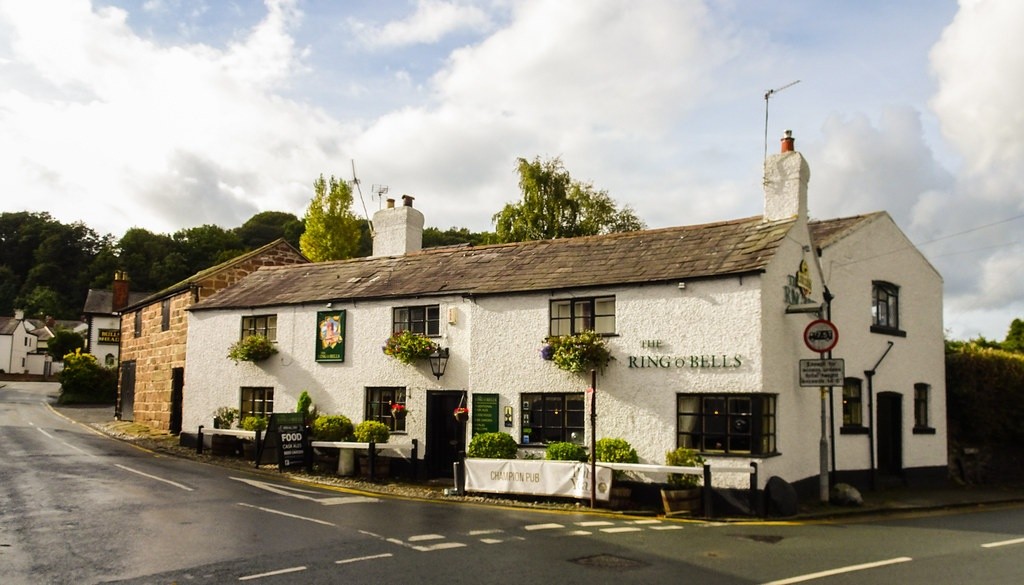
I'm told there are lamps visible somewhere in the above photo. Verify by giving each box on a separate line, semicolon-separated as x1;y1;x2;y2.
428;344;450;381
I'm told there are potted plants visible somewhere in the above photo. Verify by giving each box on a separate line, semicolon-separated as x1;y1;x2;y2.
661;446;707;515
352;420;392;478
239;413;270;459
315;415;353;472
211;406;243;457
544;441;591;503
226;332;280;367
588;437;639;510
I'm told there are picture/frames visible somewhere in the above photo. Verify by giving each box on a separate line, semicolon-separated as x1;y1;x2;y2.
315;309;346;363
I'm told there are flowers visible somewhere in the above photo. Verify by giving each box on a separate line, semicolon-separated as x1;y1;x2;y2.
454;407;469;416
391;404;409;414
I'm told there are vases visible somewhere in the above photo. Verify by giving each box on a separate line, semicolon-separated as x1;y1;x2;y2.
392;412;407;420
456;414;468;421
